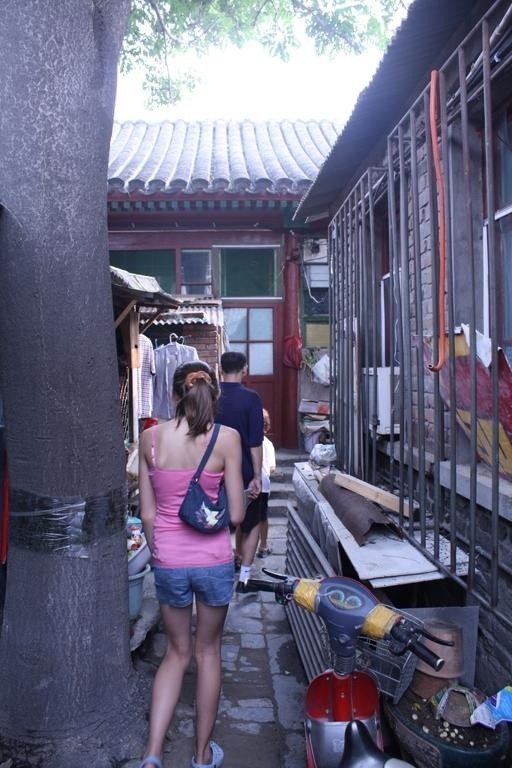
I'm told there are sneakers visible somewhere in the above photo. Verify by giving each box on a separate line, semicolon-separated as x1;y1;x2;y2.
235;580;248;593
256;545;272;558
234;558;241;573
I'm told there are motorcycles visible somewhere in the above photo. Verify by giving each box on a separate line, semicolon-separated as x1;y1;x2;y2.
235;566;456;767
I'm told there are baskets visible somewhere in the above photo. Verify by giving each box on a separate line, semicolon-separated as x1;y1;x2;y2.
318;602;425;705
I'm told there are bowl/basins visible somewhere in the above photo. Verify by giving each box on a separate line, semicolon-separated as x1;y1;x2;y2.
126;538;152;620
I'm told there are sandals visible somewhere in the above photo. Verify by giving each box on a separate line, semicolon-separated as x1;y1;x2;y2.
140;757;164;768
190;739;225;767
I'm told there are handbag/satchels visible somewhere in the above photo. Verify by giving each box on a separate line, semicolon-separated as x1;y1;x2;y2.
179;424;231;535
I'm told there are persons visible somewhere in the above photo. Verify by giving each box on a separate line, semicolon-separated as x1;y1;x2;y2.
136;358;247;767
211;351;263;594
256;406;277;559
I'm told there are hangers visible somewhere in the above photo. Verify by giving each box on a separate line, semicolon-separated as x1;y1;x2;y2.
153;334;197;354
